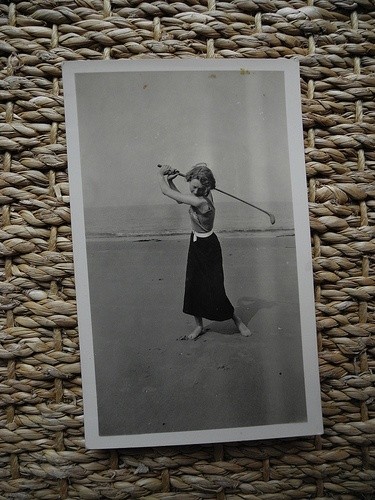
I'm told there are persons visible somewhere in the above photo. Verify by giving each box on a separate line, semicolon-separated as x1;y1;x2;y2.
159;162;253;341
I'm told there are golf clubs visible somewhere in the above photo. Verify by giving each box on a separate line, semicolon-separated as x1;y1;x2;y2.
157;162;276;224
